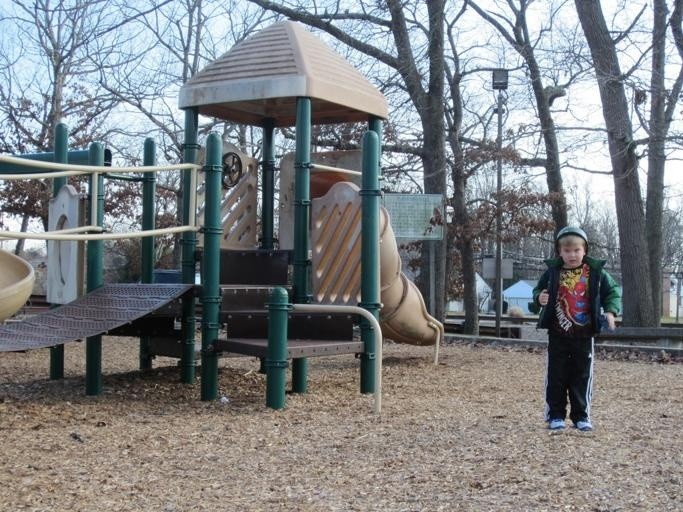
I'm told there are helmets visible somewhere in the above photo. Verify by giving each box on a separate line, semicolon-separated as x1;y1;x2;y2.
556;227;588;253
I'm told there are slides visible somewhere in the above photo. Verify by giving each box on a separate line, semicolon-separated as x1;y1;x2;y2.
0;249;35;324
380;206;444;344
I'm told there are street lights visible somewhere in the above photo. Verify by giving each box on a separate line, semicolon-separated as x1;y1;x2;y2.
491;67;510;340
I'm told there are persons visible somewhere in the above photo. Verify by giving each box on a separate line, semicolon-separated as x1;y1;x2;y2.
531;225;620;431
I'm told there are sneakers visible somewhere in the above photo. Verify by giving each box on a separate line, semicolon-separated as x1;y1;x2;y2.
549;417;566;429
574;420;593;431
